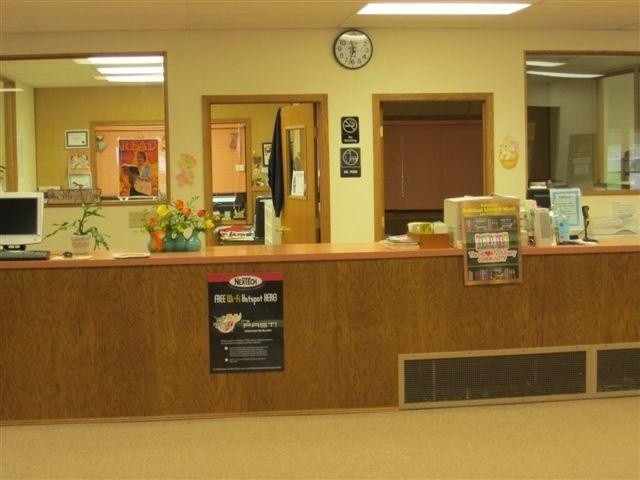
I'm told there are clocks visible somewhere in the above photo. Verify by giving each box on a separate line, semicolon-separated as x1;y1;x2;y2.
332;29;373;70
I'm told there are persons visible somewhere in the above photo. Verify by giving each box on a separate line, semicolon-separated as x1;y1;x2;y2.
122;165;152;198
123;149;152;182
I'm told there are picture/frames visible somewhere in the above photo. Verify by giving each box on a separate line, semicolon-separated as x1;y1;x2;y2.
262;144;272;166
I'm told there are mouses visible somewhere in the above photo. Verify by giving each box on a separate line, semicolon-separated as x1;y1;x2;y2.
63;252;73;257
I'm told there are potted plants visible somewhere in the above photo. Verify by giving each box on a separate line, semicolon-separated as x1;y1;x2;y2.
46;182;112;255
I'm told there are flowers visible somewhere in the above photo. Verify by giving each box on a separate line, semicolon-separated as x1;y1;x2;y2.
134;191;223;249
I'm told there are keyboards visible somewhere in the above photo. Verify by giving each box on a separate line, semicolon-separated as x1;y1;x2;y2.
1;248;50;260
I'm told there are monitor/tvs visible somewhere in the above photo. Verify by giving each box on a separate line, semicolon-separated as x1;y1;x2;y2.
1;191;44;249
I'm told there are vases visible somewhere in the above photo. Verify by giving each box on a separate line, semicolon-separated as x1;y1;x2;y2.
147;228;202;252
